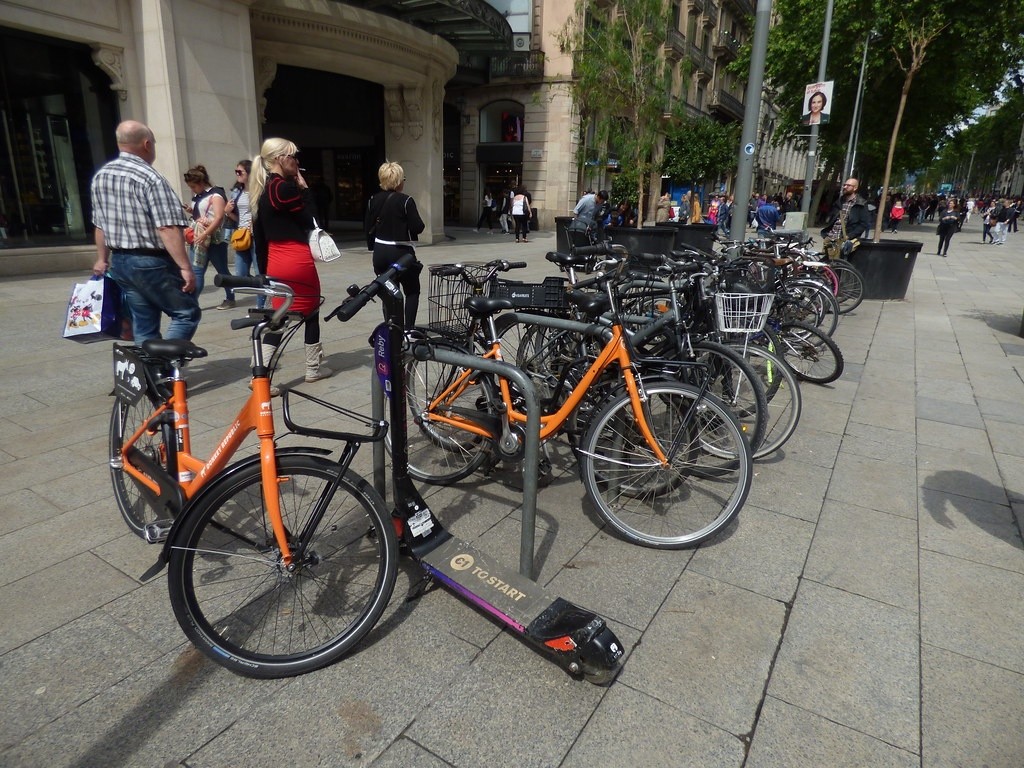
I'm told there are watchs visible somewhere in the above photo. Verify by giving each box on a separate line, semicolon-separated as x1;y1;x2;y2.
225;212;231;216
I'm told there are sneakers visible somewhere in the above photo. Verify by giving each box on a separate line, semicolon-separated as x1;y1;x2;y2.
217;300;236;310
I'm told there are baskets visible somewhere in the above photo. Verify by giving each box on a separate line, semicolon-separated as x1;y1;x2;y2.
713;292;777;334
426;262;497;340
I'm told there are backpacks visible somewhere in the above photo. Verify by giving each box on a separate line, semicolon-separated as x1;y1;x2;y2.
491;198;497;211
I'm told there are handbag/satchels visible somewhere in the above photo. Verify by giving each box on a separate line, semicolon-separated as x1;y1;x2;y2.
307;214;342;266
231;223;251;251
522;196;530;215
184;193;214;245
63;269;122;344
367;192;396;251
482;199;487;205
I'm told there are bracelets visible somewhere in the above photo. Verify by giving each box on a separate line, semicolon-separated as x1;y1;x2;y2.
204;230;209;239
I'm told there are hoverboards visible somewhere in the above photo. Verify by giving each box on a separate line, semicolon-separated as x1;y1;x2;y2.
325;250;623;688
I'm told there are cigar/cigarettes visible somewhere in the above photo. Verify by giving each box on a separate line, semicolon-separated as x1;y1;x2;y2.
298;168;305;170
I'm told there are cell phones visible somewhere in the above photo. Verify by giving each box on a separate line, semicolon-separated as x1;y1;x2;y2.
182;206;187;209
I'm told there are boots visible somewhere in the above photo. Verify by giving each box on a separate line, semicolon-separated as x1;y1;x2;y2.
305;342;333;383
248;344;281;398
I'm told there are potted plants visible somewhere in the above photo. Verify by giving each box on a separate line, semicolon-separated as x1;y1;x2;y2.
841;12;952;298
530;0;742;273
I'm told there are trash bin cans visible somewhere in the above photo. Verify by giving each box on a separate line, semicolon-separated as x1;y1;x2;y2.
554;215;577;273
529;208;538;230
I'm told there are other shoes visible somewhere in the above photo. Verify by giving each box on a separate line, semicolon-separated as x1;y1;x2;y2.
515;238;529;243
184;342;208;357
473;228;479;234
485;230;494;235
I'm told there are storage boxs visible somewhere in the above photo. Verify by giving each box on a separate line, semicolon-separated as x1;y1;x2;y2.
494;275;572;310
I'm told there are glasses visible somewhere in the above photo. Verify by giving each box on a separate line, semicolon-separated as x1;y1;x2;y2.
843;184;852;187
403;176;406;181
235;169;245;176
183;173;201;181
280;153;297;162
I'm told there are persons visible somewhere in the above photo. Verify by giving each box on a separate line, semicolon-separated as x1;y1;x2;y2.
363;157;425;330
248;137;333;396
223;160;267;318
473;179;532;243
92;120;201;348
656;190;797;240
803;92;830;125
570;190;638;229
183;165;235;310
821;179;870;281
874;192;1024;257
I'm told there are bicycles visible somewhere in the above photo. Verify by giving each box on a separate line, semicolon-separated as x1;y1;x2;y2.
408;220;870;550
103;277;391;682
